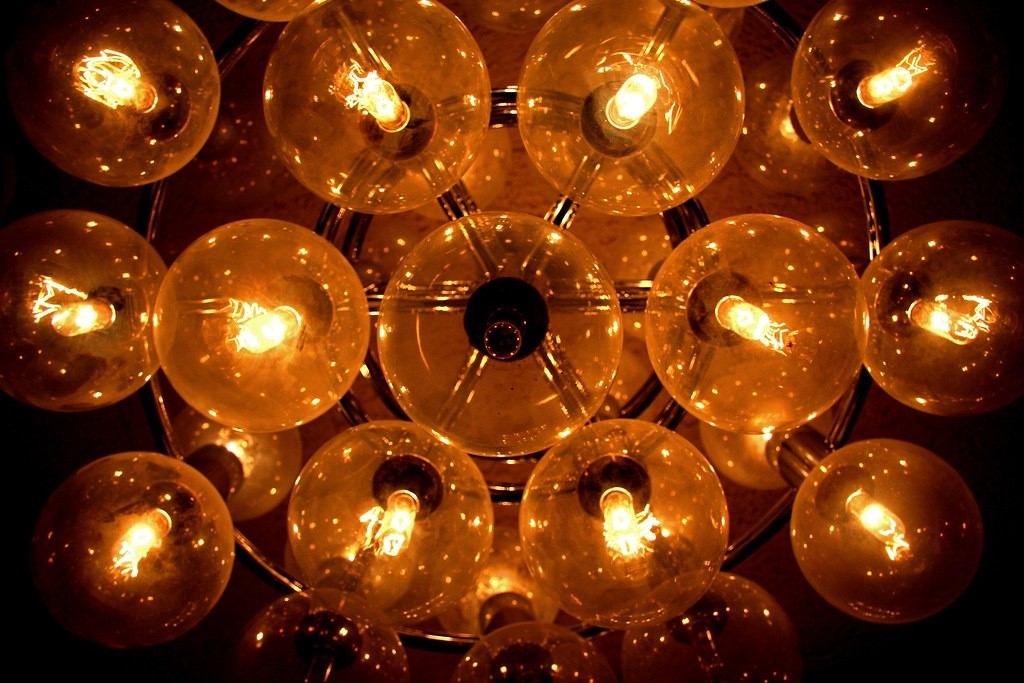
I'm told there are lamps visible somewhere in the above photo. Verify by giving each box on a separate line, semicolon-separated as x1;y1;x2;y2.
0;1;1023;683
